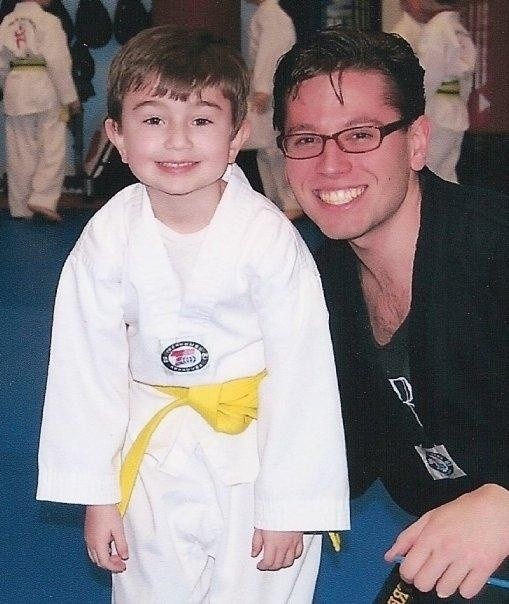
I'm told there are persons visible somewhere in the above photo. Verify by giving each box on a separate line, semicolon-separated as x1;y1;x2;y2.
1;0;83;224
32;22;351;604
391;0;427;54
416;0;477;188
236;0;306;220
266;25;509;603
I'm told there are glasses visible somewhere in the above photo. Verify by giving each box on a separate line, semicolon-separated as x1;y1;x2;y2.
274;120;411;154
